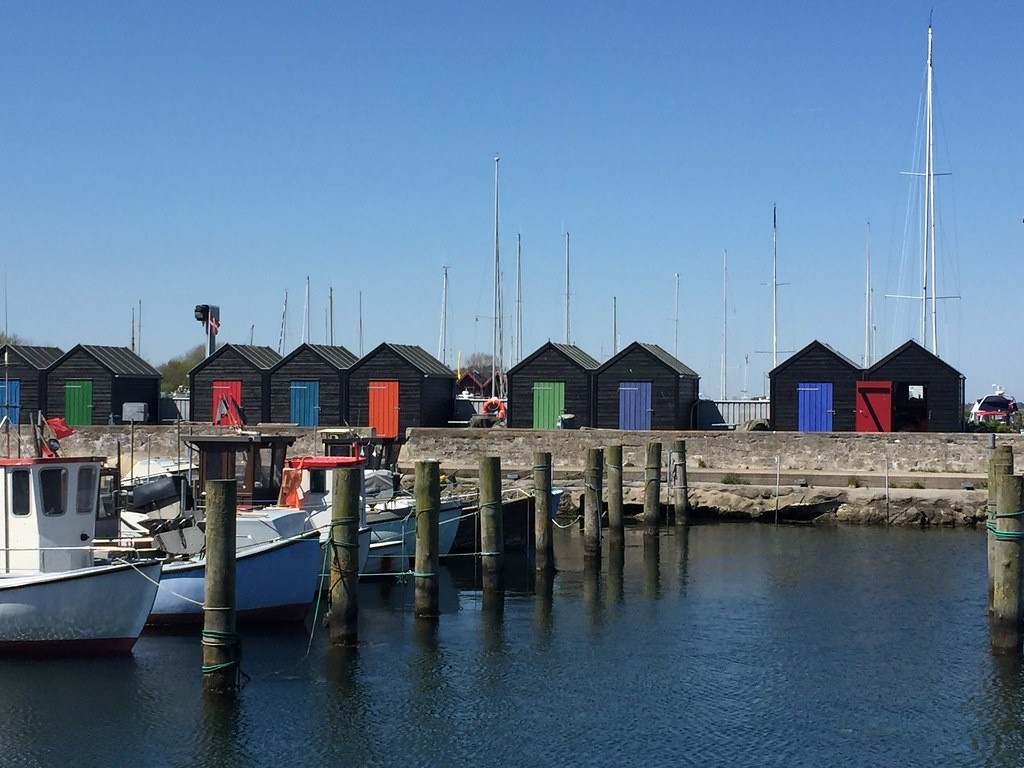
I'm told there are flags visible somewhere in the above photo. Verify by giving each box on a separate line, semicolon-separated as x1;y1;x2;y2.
46;417;76;440
209;315;221;336
215;394;248;427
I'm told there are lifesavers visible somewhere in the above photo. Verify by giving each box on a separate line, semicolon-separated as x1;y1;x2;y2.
482;399;506;420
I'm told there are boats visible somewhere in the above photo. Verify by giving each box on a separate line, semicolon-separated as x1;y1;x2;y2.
1;273;170;660
93;403;565;638
